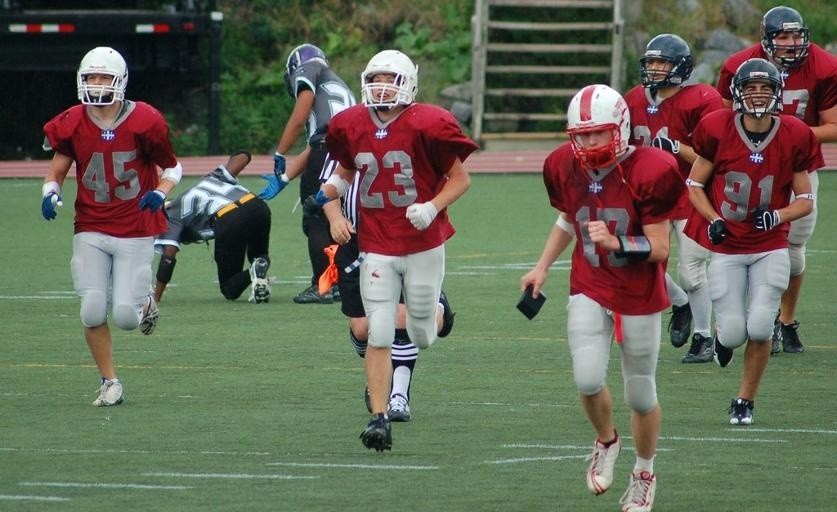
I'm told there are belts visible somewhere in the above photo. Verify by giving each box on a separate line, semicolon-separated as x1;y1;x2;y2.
217;193;255;219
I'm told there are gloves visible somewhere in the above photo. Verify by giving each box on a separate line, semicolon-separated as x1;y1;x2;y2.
752;209;780;231
139;190;166;212
41;183;64;221
302;191;332;222
258;170;288;200
708;218;727;245
650;138;681;155
405;201;437;232
274;153;286;176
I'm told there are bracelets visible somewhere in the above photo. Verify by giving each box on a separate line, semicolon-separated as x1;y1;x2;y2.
616;233;650;258
40;181;61;197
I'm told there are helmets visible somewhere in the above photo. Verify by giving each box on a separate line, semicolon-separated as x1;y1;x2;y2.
566;83;632;171
639;33;695;89
759;5;810;68
731;58;783;119
361;49;418;111
76;46;130;108
285;42;325;86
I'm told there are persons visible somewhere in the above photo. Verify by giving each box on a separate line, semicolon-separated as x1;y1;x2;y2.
258;44;356;303
318;50;480;452
716;6;837;353
622;35;724;363
40;46;182;406
689;58;826;424
151;153;271;303
519;84;689;511
318;138;420;421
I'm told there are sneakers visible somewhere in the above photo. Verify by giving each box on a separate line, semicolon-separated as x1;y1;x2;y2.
668;289;734;368
438;291;455;339
293;285;342;304
248;257;270;303
361;381;410;452
140;297;158;335
772;310;805;354
622;469;656;511
730;397;753;426
587;428;622;495
92;380;124;407
